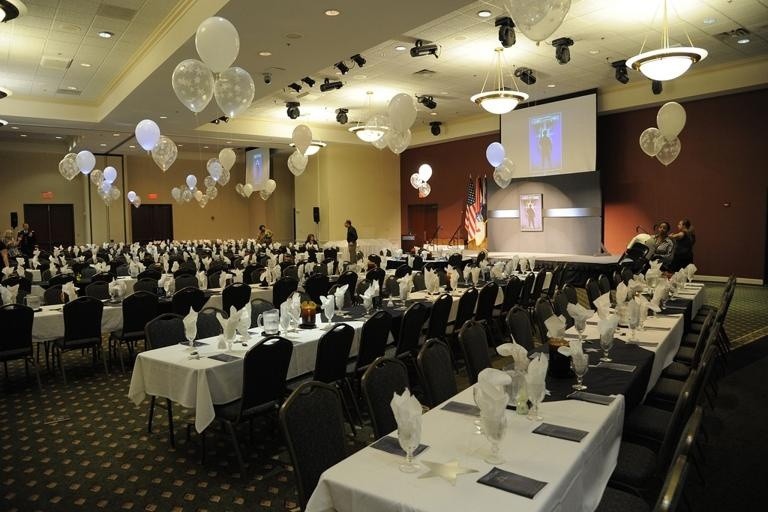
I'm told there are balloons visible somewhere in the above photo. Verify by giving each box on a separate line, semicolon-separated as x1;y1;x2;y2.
503;1;570;46
640;128;664;157
288;155;305;177
207;158;220;172
171;187;181;202
77;150;95;174
186;174;197;190
195;17;240;74
171;59;214;112
132;196;141;208
292;151;308;170
59;158;78;181
410;173;421;189
365;114;390;150
493;164;511;189
214;67;255;117
388;94;416;134
205;186;218;200
89;169;103;187
219;149;236;171
486;142;505;167
386;127;411;154
135;119;160;151
241;188;245;197
656;136;681;166
244;183;253;198
419;165;432;182
292;125;312;155
179;185;209;209
657;102;686;143
209;163;222;181
204;176;216;188
98;181;120;207
266;180;276;192
260;190;271;200
218;168;230;187
103;166;117;183
64;153;80;174
127;191;136;203
418;184;430;197
236;184;243;195
152;135;177;173
501;158;514;174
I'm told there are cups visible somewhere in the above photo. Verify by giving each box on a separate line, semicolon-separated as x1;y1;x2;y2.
291;308;301;331
185;326;197;352
363;299;372;317
506;370;525;408
280;314;291;338
257;309;280;335
600;333;614;362
23;296;40;310
627;317;640;343
324;303;334;329
647;277;657;296
399;291;408;308
335;295;345;315
163;284;169;298
521;267;526;275
472;387;483;426
472;275;478;288
571;353;589;391
218;278;226;295
479;415;508;466
300;300;316;326
127;266;139;279
427;283;436;301
687;274;693;284
398;425;422;474
118;288;125;302
574;320;587;343
530;264;535;272
223;328;237;354
450;278;458;295
525;380;546;422
163;264;169;275
463;271;470;286
109;287;115;300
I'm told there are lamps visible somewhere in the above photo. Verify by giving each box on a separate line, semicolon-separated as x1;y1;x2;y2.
416;95;436;110
285;102;300;119
551;36;574;65
211;119;221;124
514;67;536;85
348;124;388;142
625;45;708;82
410;39;439;58
429;121;442;136
0;118;9;128
651;80;662;94
291;141;325;157
494;17;516;48
301;77;316;86
0;87;13;103
611;59;629;84
0;0;27;24
288;83;302;94
221;116;229;122
350;53;366;68
333;62;349;75
320;77;343;92
469;89;530;113
336;108;350;124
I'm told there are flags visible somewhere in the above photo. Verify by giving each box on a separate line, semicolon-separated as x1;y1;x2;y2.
465;173;476;244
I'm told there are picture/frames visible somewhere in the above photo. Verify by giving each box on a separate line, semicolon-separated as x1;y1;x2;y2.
519;194;545;233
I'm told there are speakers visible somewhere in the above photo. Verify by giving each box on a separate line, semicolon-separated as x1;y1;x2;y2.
313;207;319;223
10;212;18;228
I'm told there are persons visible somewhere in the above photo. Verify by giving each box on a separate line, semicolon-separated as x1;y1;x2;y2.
257;225;266;243
538;130;552;167
525;204;535;229
669;219;695;271
260;229;273;248
651;223;673;269
17;223;38;258
305;235;318;250
1;230;19;267
345;220;358;263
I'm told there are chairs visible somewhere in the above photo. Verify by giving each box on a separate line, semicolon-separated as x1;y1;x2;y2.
417;337;457;410
284;265;298;280
91;271;116;283
683;302;730;376
477;251;486;265
196;307;228;338
613;271;621;288
429;294;459;375
449;253;462;267
531;299;556;345
506;303;535;353
598;275;611;298
137;270;162;279
505;277;533;339
324;258;338;274
286;323;360;436
702;273;730;343
688;285;732;363
692;277;735;356
542;273;558;312
222;282;251;317
338;271;358;298
552;288;572;328
459;320;493;387
468;264;476;268
585;278;600;311
396;263;412;277
367;268;386;295
81;281;112;356
623;367;704;452
306;274;330;302
478;282;506;357
413;256;422;271
277;380;351;512
522;273;535;334
286;291;313;303
7;243;393;270
252;268;268;283
698;307;730;343
413;272;426;289
175;273;199;290
563;285;578;307
356;277;372;300
51;295;110;385
3;275;31;288
345;309;393;427
359;355;408;441
108;291;159;378
329;285;353;308
273;276;298;309
4;289;34;376
199;335;294;471
44;284;71;371
532;268;546;316
209;271;236;288
48;273;76;284
145;313;196;449
394;302;427;355
172;285;204;317
422;262;433;271
456;287;478;330
661;310;718;410
612;404;703;489
133;278;159;293
0;303;45;392
593;454;688;512
249;298;277;329
622;267;632;286
651;344;718;410
386;275;399;298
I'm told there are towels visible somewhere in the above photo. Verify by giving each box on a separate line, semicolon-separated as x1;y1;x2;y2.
6;284;19;303
627;279;641;299
60;244;64;251
338;259;344;272
289;242;292;248
239;244;243;248
139;251;145;260
213;307;244;338
158;274;167;287
102;262;111;275
15;257;25;266
304;251;308;258
434;273;439;287
444;264;453;278
672;272;683;286
269;242;273;250
279;253;283;263
80;246;85;252
512;255;519;269
629;298;640;327
544;313;567;340
212;251;216;259
91;244;96;249
164;261;169;272
527;256;535;269
669;275;681;290
216;238;244;245
294;245;299;250
615;281;627;305
202;257;212;270
231;246;236;254
73;248;79;256
247;239;266;252
280;299;291;329
506;260;513;275
110;248;114;254
224;245;227;252
49;255;59;264
656;278;669;300
313;245;319;250
398;277;410;300
593;292;612;318
286;247;291;256
407;254;415;268
199;270;205;283
230;267;245;283
183;306;198;339
525;353;548;404
74;246;77;251
116;249;120;255
108;277;117;296
220;249;223;258
414;246;420;254
396;273;414;291
424;267;436;293
306;244;308;249
275;264;281;277
495;334;530;372
422;251;427;259
117;280;127;297
305;262;316;274
129;258;137;270
371;280;380;298
250;253;257;263
183;251;190;262
108;253;113;262
650;288;662;308
163;279;175;291
219;271;226;288
309;242;312;249
389;387;422;451
557;339;589;373
62;281;78;302
519;257;527;272
633;273;645;283
172;261;180;272
327;260;334;275
645;259;663;280
685;264;697;281
111;238;217;251
679;268;688;282
60;264;68;274
194;255;200;269
54;247;60;256
398;248;403;255
470;267;481;283
463;263;471;279
298;264;304;278
48;262;58;276
138;262;146;272
2;266;14;281
238;248;246;257
362;288;374;310
357;259;362;270
241;255;249;266
319;294;334;316
17;266;25;278
92;255;97;263
567;302;594;330
86;244;91;250
492;261;505;277
635;294;661;323
269;253;278;259
237;299;251;336
307;242;310;246
0;284;14;310
78;255;85;262
479;260;488;270
106;243;109;248
32;257;41;268
473;368;512;439
153;253;159;263
102;242;106;249
334;284;348;308
288;292;301;322
123;253;131;263
316;253;323;263
266;247;270;256
59;256;67;266
296;242;299;246
90;262;101;274
449;269;459;289
600;313;620;344
68;246;71;252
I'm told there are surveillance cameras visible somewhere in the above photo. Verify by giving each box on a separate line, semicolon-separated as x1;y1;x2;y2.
262;72;272;84
